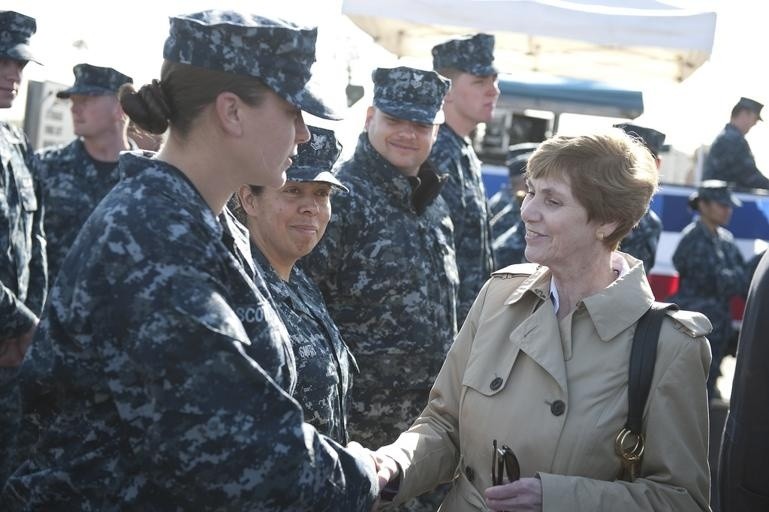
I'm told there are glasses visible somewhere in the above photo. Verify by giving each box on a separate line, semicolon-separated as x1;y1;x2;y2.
491;438;519;488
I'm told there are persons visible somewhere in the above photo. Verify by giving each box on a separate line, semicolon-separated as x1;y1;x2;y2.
701;98;769;193
429;33;501;335
240;125;362;448
0;9;380;512
487;122;666;276
31;64;140;294
365;130;712;512
664;178;744;403
297;66;460;512
1;11;47;399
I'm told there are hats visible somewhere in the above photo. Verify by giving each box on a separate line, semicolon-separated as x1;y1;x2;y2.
739;97;765;122
613;122;665;158
507;143;540;173
287;127;350;191
373;67;452;125
1;10;45;66
164;9;344;123
433;32;498;77
698;179;742;209
56;64;133;102
